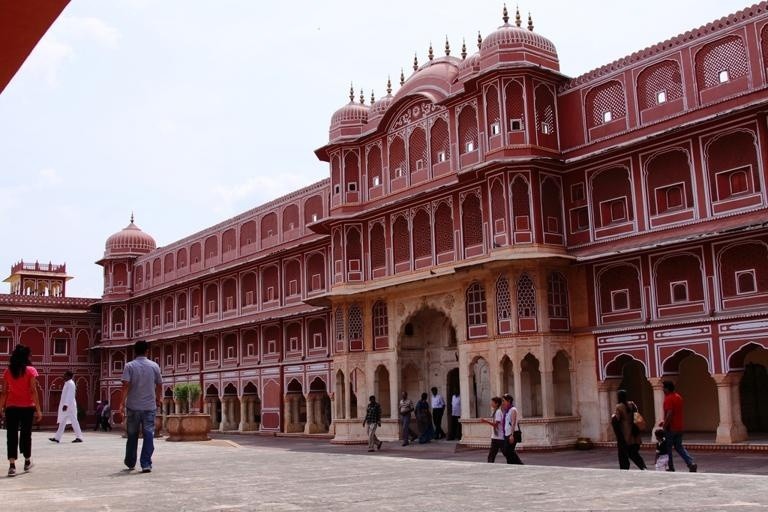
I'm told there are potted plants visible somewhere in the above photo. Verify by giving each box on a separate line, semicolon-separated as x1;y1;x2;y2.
162;381;218;443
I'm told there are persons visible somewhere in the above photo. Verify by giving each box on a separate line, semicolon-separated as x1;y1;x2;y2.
415;393;432;443
121;341;164;473
654;429;670;472
399;391;416;446
1;344;42;476
446;387;461;441
431;387;446;440
49;371;83;443
501;393;523;464
662;380;697;472
611;390;648;470
94;400;112;432
363;396;382;452
480;397;510;463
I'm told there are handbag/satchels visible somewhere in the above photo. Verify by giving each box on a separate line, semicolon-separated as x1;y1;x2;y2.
503;430;522;444
633;411;650;433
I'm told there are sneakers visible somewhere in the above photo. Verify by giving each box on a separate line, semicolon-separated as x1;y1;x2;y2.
368;449;375;453
141;467;152;473
666;467;676;471
377;441;382;449
7;467;16;478
129;466;135;470
24;462;34;471
49;438;59;444
402;434;462;446
688;462;697;472
72;438;82;442
